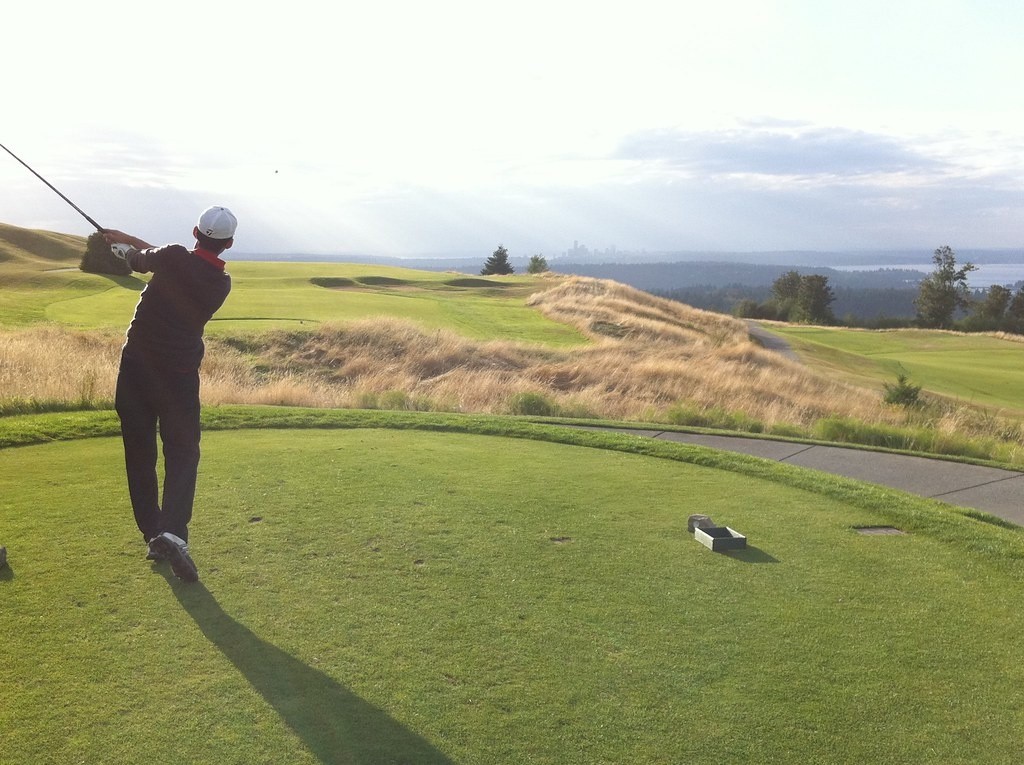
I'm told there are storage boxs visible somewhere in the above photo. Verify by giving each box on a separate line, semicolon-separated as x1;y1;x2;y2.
694;526;746;551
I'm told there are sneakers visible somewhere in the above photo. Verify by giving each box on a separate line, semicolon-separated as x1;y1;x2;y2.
146;532;198;582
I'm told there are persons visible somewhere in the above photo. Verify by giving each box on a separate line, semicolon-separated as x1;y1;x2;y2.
100;206;238;583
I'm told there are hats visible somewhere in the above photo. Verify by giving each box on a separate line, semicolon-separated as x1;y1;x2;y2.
198;205;238;240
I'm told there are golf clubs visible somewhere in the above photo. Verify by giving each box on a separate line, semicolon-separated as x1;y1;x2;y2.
0;143;117;244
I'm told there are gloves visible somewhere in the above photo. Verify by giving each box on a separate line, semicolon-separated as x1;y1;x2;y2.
111;241;137;260
